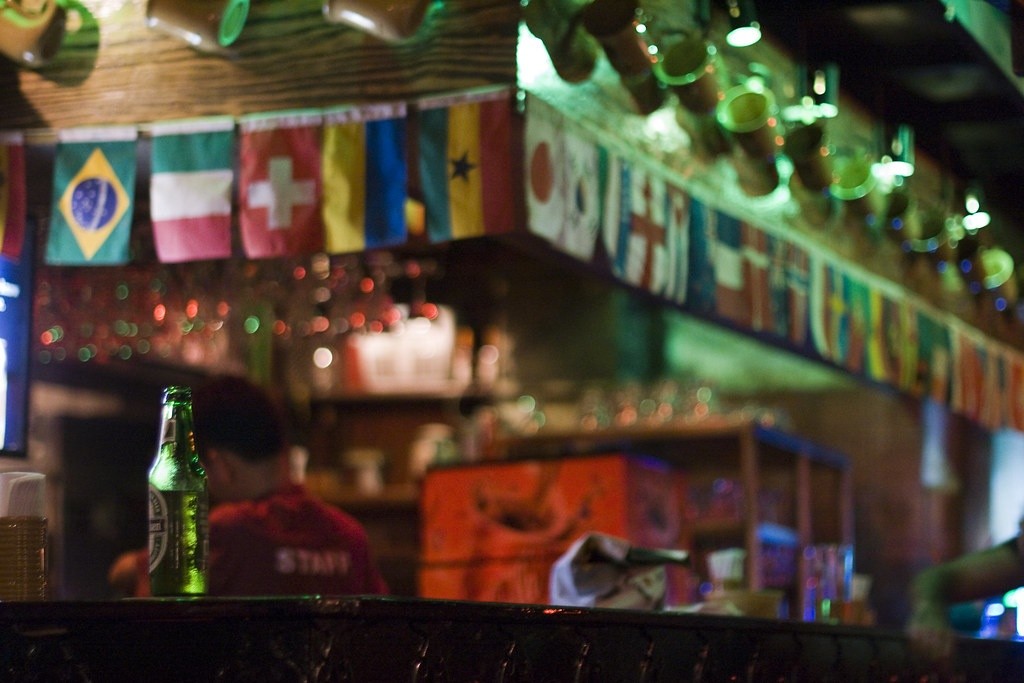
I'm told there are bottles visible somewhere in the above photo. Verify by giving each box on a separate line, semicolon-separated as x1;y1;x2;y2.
146;384;209;595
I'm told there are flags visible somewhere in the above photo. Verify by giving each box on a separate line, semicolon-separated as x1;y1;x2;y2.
149;115;234;264
415;83;516;246
0;132;27;261
238;100;409;258
44;126;137;268
524;87;1023;432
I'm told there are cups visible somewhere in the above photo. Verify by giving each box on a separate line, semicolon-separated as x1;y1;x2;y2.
801;543;855;625
0;516;48;602
0;0;68;72
324;0;441;47
581;0;1018;310
145;1;248;53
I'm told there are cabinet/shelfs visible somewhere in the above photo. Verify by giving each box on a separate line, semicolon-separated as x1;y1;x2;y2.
426;421;851;621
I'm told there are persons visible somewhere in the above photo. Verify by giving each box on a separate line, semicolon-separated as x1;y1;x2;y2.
103;375;392;595
906;518;1024;661
